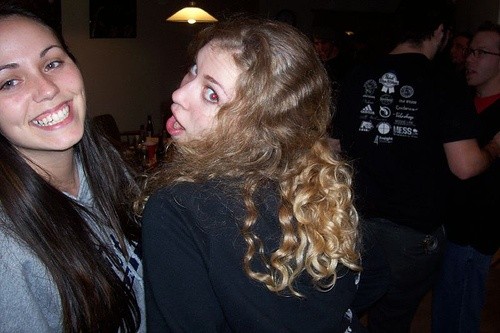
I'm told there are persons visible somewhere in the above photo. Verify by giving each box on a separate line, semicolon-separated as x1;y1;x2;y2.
327;0;500;333
138;20;366;333
0;0;145;333
445;30;473;85
312;28;361;136
431;23;500;333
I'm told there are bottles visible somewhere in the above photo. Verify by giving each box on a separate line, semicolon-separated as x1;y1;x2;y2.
140;114;173;158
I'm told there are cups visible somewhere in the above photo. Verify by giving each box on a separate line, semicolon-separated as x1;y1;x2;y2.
144;142;157;170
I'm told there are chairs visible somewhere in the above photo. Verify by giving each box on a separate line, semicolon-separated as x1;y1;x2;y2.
91;114;147;167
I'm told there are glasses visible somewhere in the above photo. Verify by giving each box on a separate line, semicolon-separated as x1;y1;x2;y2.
462;47;500;58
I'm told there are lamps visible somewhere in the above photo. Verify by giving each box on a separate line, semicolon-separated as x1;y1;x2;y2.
166;0;218;25
